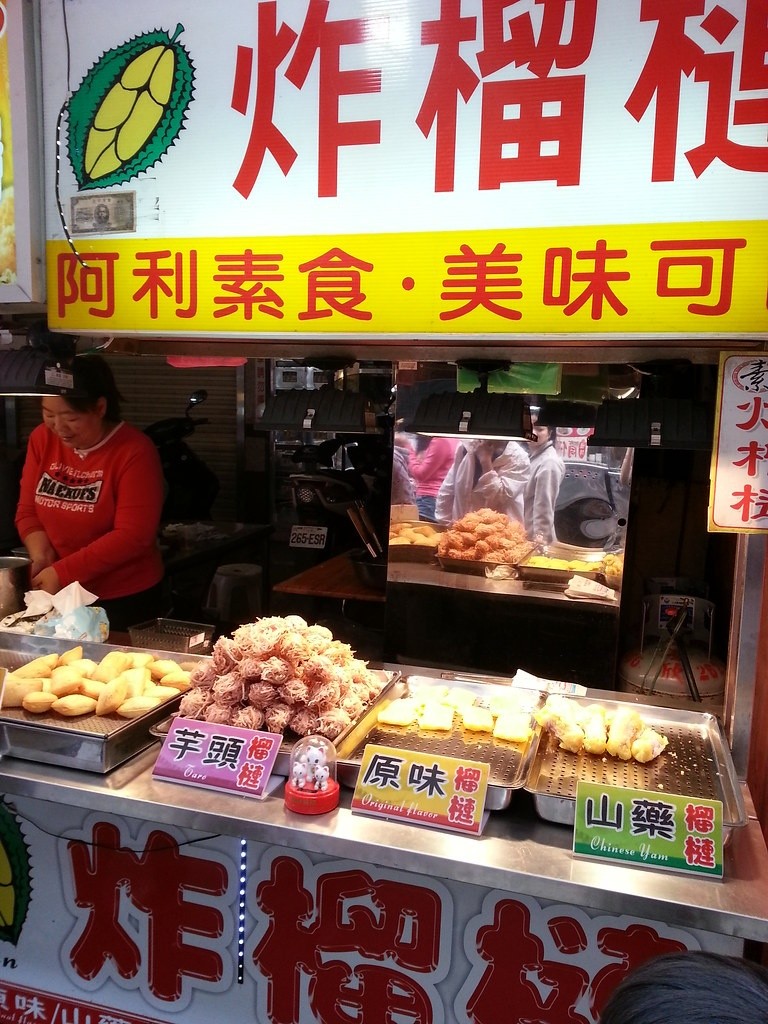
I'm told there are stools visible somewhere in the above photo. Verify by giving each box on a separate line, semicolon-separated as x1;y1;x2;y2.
205;565;263;622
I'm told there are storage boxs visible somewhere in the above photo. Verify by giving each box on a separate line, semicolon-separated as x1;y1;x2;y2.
128;618;215;655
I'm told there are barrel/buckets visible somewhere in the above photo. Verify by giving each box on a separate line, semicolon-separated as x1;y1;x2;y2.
0;556;34;623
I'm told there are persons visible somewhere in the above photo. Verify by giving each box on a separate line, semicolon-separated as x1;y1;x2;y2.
396;421;565;544
15;351;175;634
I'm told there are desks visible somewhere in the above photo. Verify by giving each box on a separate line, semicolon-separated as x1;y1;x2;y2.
272;549;386;619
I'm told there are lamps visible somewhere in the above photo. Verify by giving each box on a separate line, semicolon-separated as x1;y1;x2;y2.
1;326;64;398
